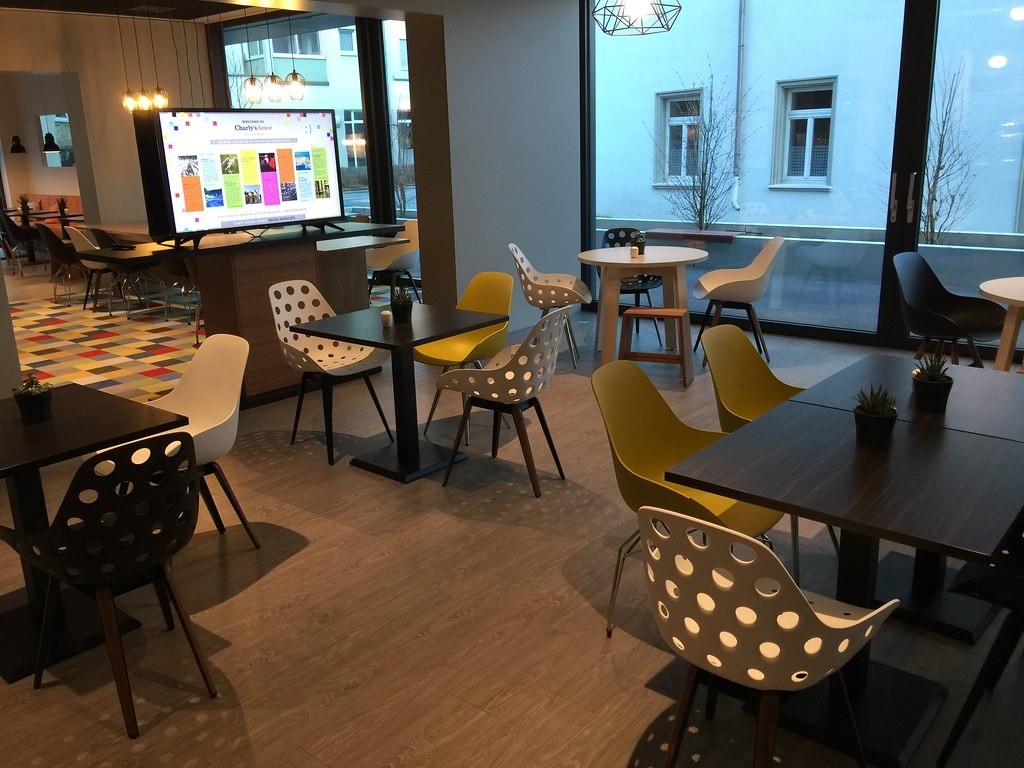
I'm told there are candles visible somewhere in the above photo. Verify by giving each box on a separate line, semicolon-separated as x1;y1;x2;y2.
17;207;21;213
64;209;69;215
380;310;393;328
631;246;638;258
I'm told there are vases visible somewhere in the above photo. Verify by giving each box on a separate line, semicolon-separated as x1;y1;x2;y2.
13;388;53;425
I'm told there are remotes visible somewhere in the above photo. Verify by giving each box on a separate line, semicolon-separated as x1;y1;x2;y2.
109;244;136;248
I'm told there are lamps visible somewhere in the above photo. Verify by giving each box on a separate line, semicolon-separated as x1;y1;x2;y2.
591;0;680;36
242;5;263;103
9;134;27;154
285;10;306;101
263;7;284;103
130;3;152;111
146;0;169;110
39;78;61;154
114;5;137;116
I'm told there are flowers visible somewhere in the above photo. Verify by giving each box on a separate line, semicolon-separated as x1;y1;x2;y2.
12;372;51;395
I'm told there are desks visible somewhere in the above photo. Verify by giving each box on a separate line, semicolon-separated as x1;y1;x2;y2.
576;244;709;371
28;212;82;240
77;228;295;320
0;383;189;685
978;276;1024;372
788;352;1024;646
288;304;510;485
5;210;57;266
75;219;256;291
60;215;85;222
665;398;1024;768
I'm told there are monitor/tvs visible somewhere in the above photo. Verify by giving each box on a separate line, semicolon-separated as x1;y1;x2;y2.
134;108;345;243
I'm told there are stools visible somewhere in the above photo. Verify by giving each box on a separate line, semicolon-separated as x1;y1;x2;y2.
617;308;695;388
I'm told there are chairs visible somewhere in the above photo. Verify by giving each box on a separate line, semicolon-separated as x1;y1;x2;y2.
892;251;1009;369
98;335;261;568
691;235;786;368
435;305;571;501
636;504;902;768
365;219;422;304
413;270;513;447
594;228;664;348
268;280;392;465
0;208;203;348
0;431;219;740
507;242;592;369
591;358;787;641
700;324;840;555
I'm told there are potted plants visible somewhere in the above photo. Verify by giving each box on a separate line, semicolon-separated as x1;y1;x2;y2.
56;194;68;215
851;383;899;445
389;287;413;323
15;193;29;212
911;353;955;408
630;230;646;254
